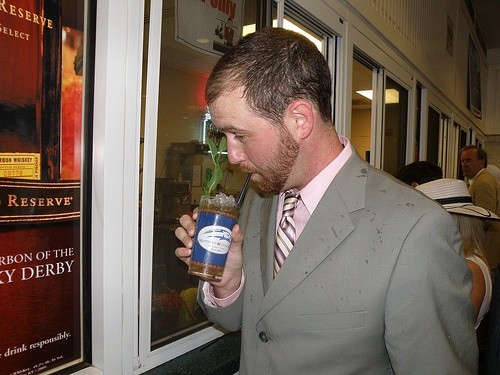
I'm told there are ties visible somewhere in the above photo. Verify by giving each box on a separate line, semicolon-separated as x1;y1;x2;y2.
272;190;300;280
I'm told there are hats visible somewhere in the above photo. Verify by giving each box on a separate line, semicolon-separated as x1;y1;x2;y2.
415;179;500;221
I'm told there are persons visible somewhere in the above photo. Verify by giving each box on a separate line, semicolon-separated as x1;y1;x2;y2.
176;28;479;375
396;144;498;330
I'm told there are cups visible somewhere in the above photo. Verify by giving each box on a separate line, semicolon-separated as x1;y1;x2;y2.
187;194;239;283
